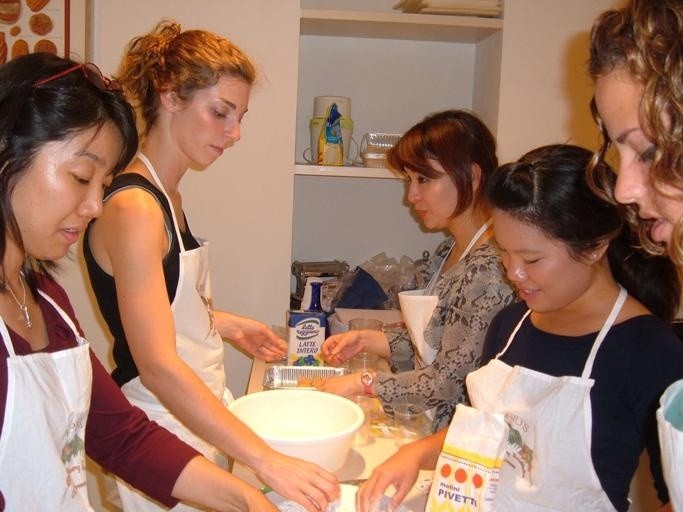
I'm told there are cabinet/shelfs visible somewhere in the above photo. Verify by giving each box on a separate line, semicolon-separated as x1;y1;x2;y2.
291;6;503;179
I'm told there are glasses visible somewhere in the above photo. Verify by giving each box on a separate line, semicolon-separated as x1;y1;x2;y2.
30;61;124;93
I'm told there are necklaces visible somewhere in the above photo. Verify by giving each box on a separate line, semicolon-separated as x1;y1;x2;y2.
6;276;34;330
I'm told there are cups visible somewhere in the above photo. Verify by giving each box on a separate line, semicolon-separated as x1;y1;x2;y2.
349;318;383;362
394;403;425;447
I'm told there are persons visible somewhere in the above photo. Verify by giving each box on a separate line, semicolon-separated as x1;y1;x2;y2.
41;20;342;512
0;53;280;512
313;110;523;433
581;1;683;512
354;141;683;512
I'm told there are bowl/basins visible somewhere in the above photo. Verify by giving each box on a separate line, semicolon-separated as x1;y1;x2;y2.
224;388;365;474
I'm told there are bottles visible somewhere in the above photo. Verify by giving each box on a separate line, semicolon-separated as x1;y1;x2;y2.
307;282;330;340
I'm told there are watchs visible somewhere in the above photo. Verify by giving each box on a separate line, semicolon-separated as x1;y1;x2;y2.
361;369;375;398
